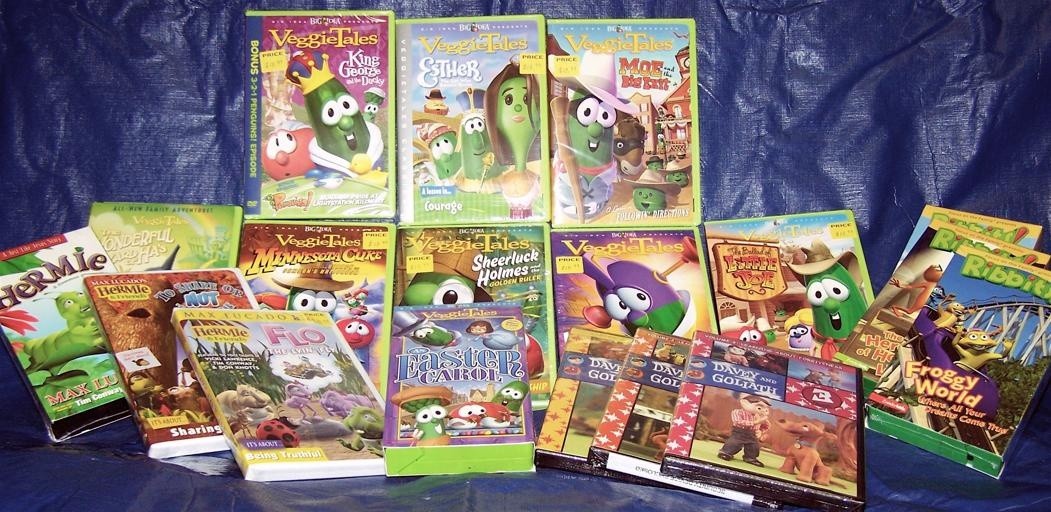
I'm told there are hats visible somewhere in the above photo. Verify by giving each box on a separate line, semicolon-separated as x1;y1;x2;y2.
271;258;355;292
548;47;640;115
425;89;446;100
396;240;493;302
785;240;863;288
